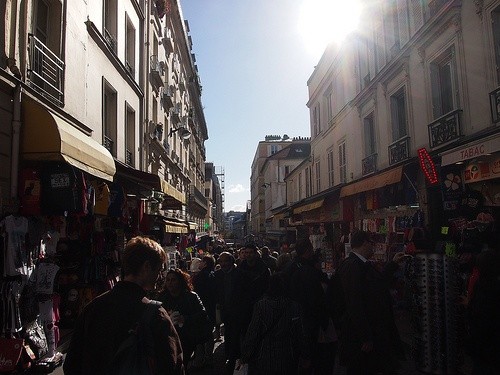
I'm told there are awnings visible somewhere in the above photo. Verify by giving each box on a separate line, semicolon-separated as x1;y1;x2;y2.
113;164;186;207
19;98;116;182
273;210;290;221
439;133;500;167
144;212;209;239
339;164;407;199
294;197;324;214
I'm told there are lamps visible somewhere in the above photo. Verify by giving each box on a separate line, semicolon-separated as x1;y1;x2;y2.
171;126;192;139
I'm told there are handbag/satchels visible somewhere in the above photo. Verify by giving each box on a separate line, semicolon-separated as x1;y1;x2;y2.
234;359;249;375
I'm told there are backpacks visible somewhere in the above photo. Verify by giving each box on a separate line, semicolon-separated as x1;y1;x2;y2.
108;300;163;375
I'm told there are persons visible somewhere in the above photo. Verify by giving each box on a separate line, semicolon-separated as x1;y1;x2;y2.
157;267;207;374
185;230;500;375
60;235;186;375
